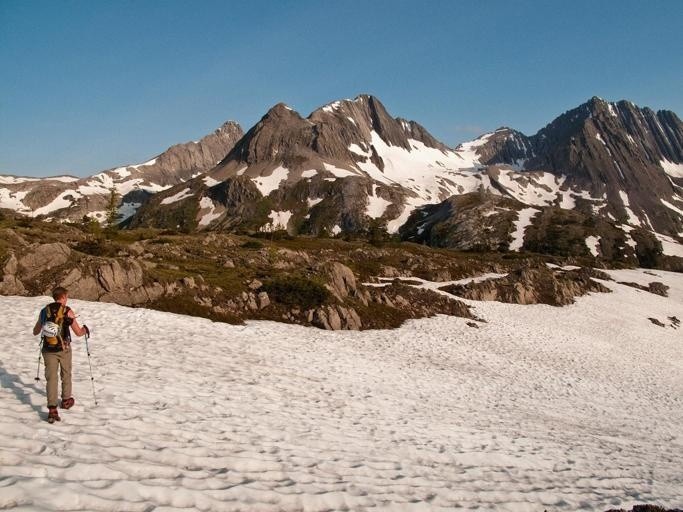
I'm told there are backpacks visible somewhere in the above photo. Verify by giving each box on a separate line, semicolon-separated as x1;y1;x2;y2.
40;302;65;352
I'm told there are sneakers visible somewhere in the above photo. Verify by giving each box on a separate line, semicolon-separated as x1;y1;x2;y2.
48;409;60;423
62;399;74;408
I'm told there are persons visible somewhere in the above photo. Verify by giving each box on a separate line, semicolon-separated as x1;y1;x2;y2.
31;285;89;424
